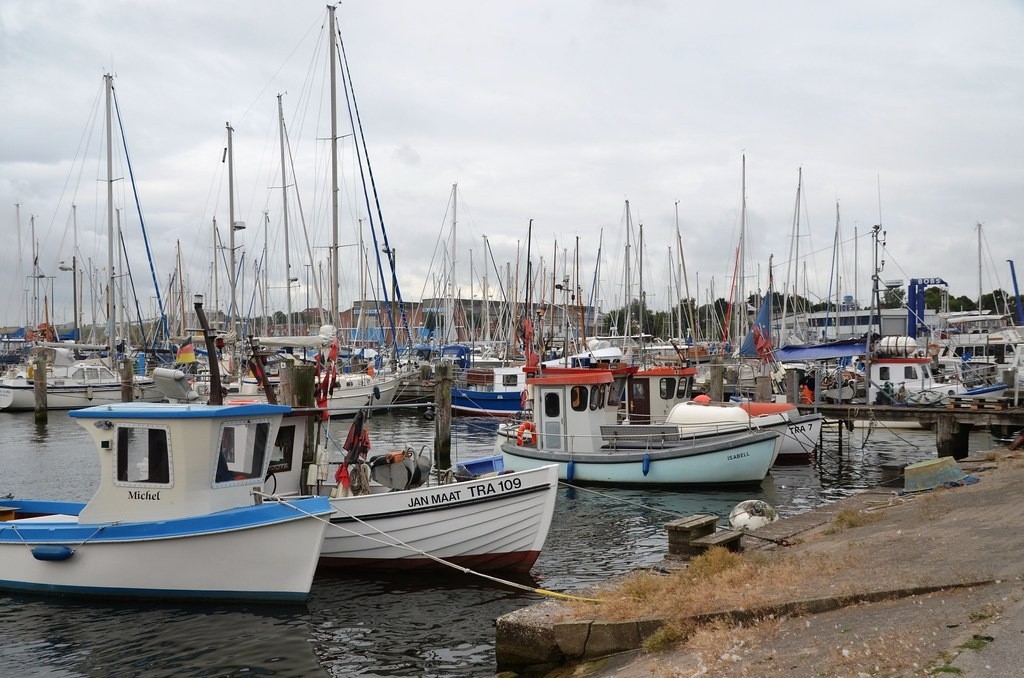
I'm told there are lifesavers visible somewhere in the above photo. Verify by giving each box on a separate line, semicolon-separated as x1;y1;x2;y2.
926;343;941;356
516;420;539;448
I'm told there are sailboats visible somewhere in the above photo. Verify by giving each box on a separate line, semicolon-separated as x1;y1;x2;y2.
0;1;1024;424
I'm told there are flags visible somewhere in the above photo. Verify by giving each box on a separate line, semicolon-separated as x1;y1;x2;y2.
733;289;771;361
177;335;196;363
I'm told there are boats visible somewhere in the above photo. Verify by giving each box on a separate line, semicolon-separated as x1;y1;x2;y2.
0;400;336;610
497;364;780;491
318;366;558;581
618;365;825;465
